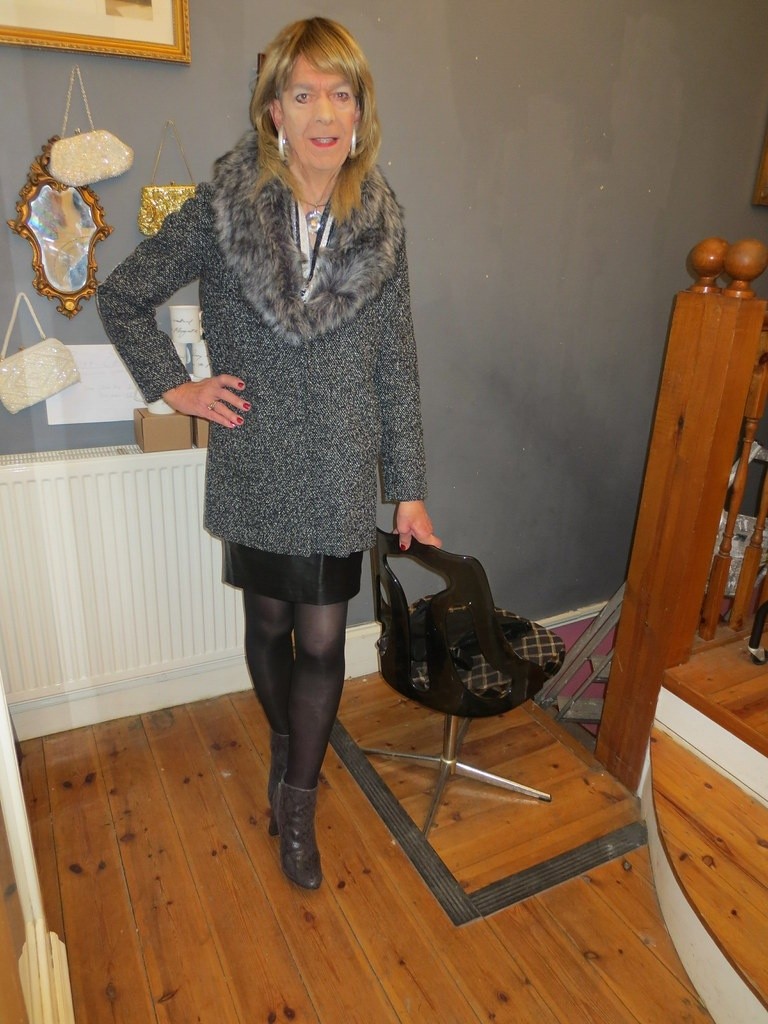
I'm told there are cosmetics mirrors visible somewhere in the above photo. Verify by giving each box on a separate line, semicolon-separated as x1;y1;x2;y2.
5;134;116;321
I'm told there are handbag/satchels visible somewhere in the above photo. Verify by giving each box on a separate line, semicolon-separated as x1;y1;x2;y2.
138;118;199;236
48;65;135;188
0;292;81;416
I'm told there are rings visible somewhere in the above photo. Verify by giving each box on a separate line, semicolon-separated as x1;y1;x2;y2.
207;399;219;411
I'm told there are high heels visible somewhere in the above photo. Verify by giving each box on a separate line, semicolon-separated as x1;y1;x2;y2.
268;777;323;890
263;728;289;805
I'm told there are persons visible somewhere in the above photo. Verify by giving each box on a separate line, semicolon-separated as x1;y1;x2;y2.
94;17;443;891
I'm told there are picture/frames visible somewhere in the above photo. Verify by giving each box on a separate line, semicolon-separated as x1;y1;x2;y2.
0;0;192;67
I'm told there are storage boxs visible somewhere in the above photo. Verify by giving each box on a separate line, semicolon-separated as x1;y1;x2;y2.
192;415;209;448
132;407;193;455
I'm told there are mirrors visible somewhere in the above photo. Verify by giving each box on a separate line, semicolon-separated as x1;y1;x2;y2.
7;133;115;320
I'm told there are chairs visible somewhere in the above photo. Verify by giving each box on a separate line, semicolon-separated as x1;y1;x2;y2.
360;526;564;844
